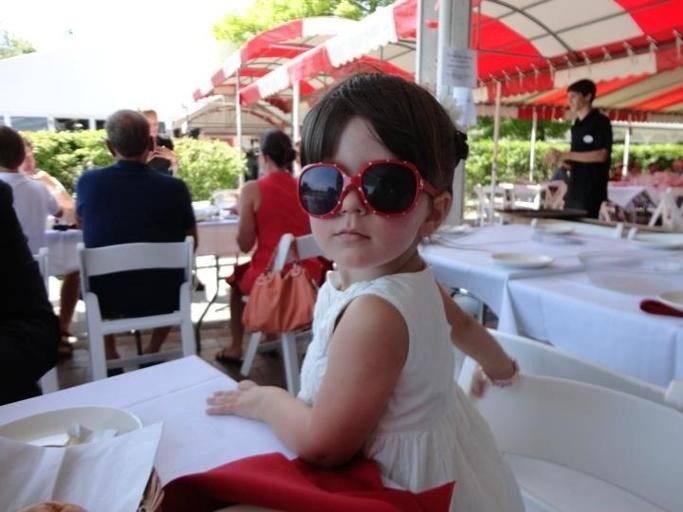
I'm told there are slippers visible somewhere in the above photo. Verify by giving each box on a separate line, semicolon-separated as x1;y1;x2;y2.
215;348;243;366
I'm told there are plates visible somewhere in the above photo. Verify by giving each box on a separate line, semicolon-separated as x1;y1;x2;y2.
1;405;141;447
492;251;555;270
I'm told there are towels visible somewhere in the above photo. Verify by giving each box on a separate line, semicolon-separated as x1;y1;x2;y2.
158;452;456;511
638;297;681;319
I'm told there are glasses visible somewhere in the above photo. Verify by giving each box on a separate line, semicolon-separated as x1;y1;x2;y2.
296;158;441;220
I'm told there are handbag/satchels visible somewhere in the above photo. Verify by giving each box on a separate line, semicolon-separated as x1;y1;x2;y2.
241;233;317;333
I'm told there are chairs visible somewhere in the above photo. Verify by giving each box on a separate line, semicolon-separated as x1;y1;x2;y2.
210;189;242;208
466;368;682;511
471;175;682;221
28;247;60;397
528;217;624;240
627;227;682;246
241;231;332;400
76;235;198;382
485;326;682;412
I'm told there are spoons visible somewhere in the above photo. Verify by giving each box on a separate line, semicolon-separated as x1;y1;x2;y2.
640;299;683;318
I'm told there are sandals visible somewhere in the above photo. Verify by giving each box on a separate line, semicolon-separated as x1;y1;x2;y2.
59;330;73;353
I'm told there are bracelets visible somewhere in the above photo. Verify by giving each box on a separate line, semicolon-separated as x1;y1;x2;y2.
477;357;519;387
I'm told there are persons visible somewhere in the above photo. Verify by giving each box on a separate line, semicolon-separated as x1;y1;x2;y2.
75;109;198;378
609;159;683;226
0;125;63;276
544;79;612;221
203;73;525;511
140;109;173;174
213;128;325;368
549;160;570;192
15;137;81;351
0;180;60;407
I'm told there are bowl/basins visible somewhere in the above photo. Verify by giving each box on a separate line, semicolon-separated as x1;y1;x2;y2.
577;249;681;293
